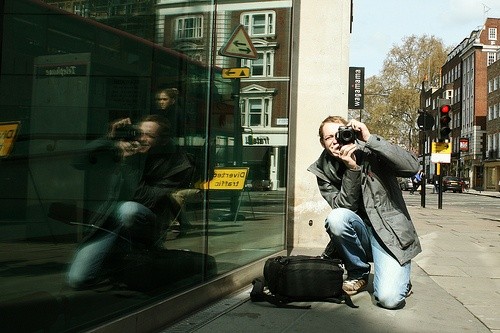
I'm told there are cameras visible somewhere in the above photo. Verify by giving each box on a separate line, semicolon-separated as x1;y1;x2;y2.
116;126;144;141
335;128;360;146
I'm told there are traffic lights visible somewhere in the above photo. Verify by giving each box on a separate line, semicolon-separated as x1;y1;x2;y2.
437;98;452;140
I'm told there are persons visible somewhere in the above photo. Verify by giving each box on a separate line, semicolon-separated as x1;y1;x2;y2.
68;114;183;291
152;88;189;236
307;114;421;308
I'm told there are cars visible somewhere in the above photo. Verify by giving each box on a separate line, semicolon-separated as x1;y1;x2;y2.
442;175;463;194
400;179;414;191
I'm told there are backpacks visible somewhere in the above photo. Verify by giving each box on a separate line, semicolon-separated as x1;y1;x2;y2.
134;245;220;295
250;254;359;310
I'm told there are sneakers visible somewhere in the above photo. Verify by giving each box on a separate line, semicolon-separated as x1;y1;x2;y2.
341;277;367;296
